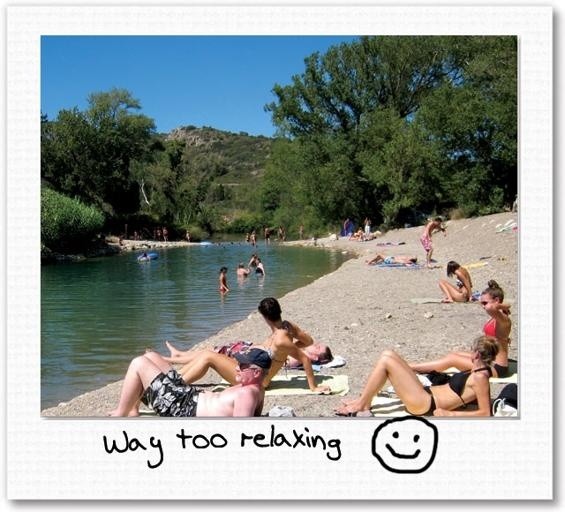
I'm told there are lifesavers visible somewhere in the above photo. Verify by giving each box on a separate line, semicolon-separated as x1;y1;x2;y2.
138;253;157;262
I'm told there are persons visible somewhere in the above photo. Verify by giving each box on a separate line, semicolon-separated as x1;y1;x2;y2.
364;253;418;267
218;266;231;294
109;347;273;416
333;335;500;417
132;214;373;246
248;254;262;267
254;257;266;277
407;279;513;378
145;337;334;372
420;217;447;270
176;297;333;394
137;253;151;263
437;261;475;304
236;263;251;280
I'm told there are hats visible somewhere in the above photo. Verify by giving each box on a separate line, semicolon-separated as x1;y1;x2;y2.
235;348;271;368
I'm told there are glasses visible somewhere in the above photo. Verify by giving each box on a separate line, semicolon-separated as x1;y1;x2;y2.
481;301;489;306
240;362;258;370
